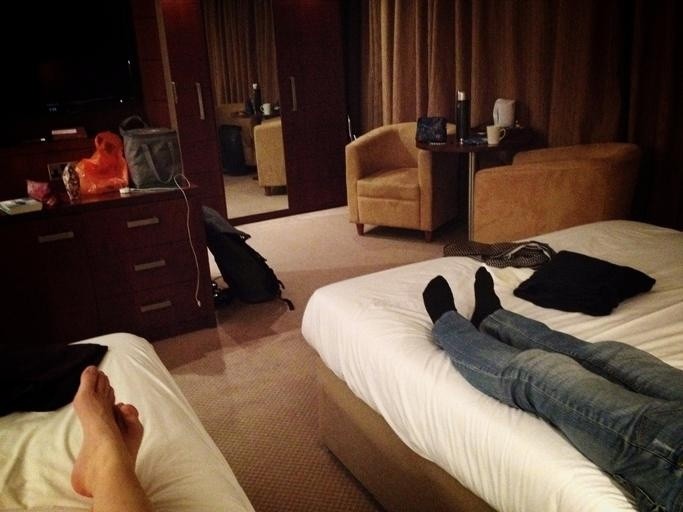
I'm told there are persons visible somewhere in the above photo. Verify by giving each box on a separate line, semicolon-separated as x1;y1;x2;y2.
423;265;683;512
65;363;154;512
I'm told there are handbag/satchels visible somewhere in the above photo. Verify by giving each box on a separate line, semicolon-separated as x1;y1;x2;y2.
415;117;448;143
119;115;181;188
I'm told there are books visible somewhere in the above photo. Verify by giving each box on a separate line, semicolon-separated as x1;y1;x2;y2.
0;199;42;214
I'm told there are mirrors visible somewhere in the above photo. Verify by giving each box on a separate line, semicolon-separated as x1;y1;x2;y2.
201;1;291;221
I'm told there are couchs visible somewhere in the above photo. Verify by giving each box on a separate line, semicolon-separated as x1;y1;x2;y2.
253;116;287;195
472;143;640;246
346;121;457;242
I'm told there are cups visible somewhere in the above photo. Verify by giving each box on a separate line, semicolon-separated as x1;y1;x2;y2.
260;103;271;116
487;125;505;144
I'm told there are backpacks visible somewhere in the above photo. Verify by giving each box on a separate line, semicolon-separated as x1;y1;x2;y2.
203;205;295;312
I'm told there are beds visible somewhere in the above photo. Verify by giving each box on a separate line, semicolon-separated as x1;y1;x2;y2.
301;219;683;511
1;333;255;512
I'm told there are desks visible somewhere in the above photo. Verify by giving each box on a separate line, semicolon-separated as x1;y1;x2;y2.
416;133;544;241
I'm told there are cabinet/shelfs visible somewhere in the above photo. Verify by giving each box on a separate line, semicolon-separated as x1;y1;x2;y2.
0;186;218;381
136;1;348;228
